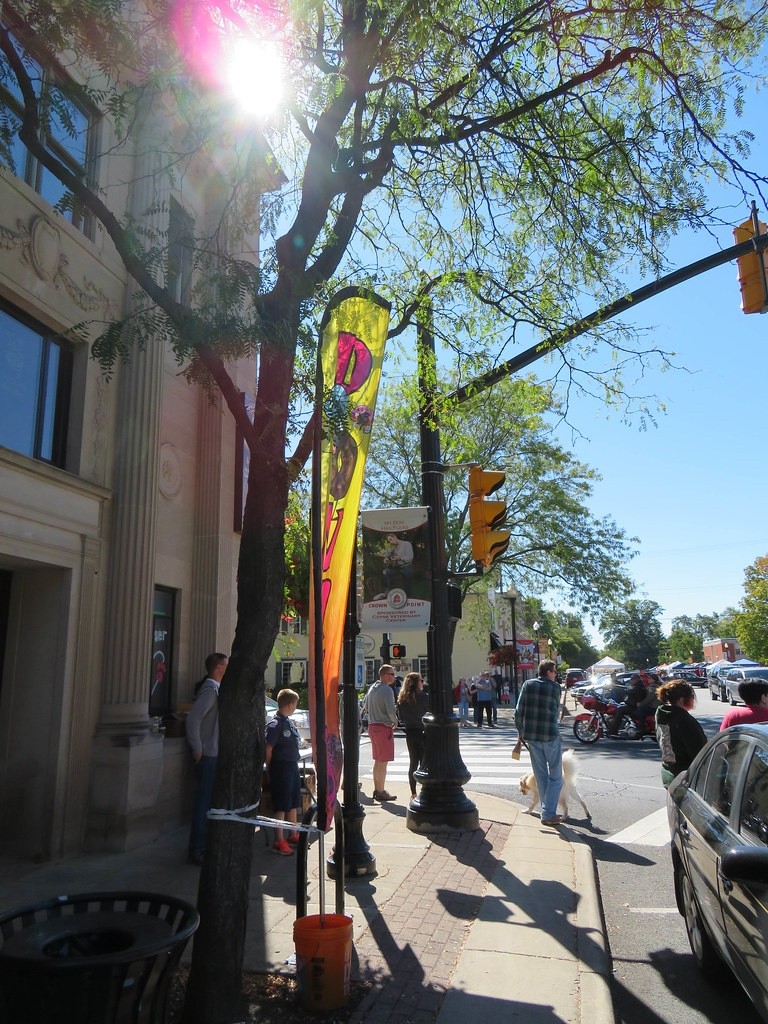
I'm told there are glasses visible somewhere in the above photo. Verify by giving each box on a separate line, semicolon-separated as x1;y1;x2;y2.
418;679;424;683
386;673;394;676
483;675;486;676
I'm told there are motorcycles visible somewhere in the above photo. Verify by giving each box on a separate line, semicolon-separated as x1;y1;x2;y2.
573;688;658;744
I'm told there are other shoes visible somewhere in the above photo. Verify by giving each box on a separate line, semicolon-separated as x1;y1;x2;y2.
187;846;205;865
488;722;494;727
477;724;482;729
541;815;560;825
606;729;618;735
460;719;468;725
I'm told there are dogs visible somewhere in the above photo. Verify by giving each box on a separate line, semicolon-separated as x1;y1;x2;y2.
518;748;593;822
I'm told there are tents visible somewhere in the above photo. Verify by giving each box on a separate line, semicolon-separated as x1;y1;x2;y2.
646;659;761;676
592;656;625;675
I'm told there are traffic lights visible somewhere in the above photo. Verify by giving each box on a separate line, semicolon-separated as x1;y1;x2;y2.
392;645;406;657
468;465;512;575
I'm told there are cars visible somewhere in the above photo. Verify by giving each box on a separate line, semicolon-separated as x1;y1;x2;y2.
724;666;768;707
667;722;768;1024
707;665;742;702
566;659;710;703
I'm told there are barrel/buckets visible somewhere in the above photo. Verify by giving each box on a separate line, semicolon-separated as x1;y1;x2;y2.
294;914;353;1011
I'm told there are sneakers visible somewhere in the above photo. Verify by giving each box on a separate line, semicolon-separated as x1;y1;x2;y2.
373;789;397;800
359;792;374;805
288;833;310;847
272;839;294;855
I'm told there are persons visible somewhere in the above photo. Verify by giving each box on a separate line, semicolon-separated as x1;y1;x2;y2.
392;679;403;698
655;678;709;790
719;676;768;732
365;664;398;801
475;671;495;727
469;676;478;724
383;533;414;597
395;672;430;801
602;671;648;736
454;676;472;728
635;672;663;735
502;680;511;705
488;668;504;705
338;687;362;791
264;688;311;855
423;676;428;692
514;659;562;824
186;652;229;867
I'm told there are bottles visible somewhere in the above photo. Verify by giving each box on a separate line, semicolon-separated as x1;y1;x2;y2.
152;716;159;732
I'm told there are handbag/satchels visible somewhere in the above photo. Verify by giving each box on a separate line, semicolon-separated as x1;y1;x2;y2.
467;694;471;703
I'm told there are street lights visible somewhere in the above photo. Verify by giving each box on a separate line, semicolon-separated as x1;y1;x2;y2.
547;638;553;660
725;642;729;661
501;578;520;708
532;619;541;664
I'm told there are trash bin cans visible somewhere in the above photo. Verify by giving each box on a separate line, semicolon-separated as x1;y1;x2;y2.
0;890;199;1024
